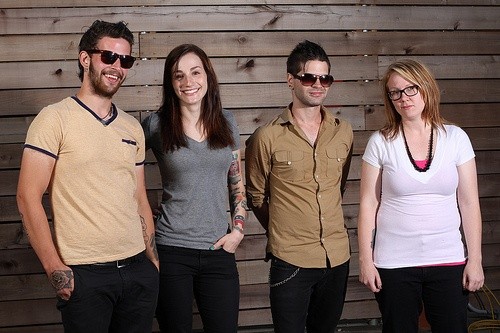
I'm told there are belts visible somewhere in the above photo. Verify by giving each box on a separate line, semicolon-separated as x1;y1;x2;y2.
95;250;146;269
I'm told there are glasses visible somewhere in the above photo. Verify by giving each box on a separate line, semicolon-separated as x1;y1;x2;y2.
293;74;334;88
87;48;136;69
387;85;419;101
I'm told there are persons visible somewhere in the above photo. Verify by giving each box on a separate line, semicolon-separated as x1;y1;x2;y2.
141;44;247;333
16;20;159;333
244;40;354;333
357;59;485;333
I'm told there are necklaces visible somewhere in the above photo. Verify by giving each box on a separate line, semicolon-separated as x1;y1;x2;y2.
75;93;113;119
400;122;434;172
